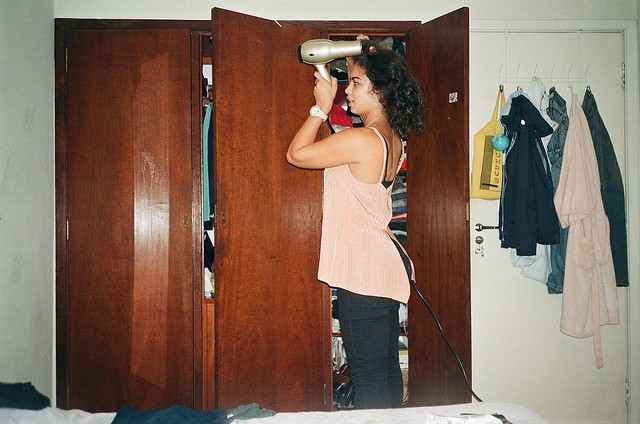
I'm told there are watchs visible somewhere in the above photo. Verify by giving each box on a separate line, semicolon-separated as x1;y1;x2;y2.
309;105;329;123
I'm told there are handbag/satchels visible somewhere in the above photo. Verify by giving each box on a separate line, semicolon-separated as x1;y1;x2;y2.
470;86;507;201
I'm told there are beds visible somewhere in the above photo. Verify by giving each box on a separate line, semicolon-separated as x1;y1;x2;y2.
1;402;541;423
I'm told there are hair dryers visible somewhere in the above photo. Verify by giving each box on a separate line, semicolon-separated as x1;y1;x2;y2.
297;37;363;86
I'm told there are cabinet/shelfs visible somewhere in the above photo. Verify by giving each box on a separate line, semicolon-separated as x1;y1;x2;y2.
54;5;325;411
321;6;472;412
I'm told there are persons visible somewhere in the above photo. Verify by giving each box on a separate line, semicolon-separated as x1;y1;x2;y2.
285;41;426;410
